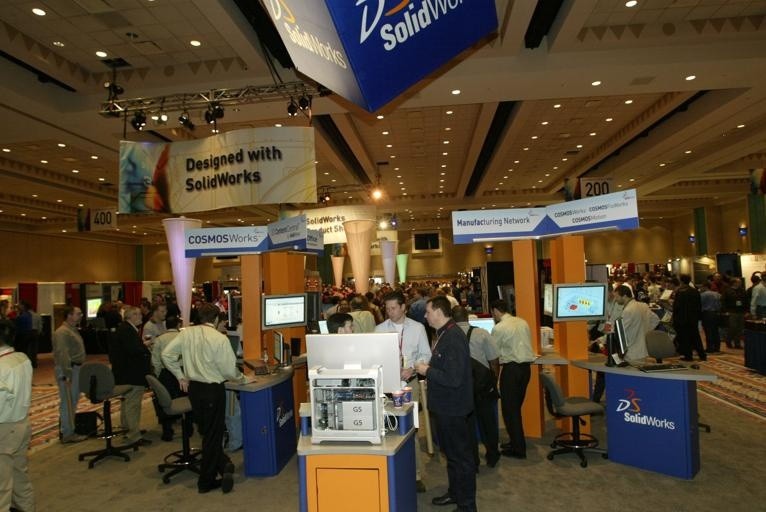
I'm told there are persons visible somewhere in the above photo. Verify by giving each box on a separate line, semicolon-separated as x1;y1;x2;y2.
366;292;384;325
142;303;167;341
326;296;340;316
706;275;713;289
0;300;9;320
347;296;375;332
750;271;766;321
374;291;431;492
151;316;194;442
700;281;722;353
321;280;355;299
25;303;42;368
607;264;679;304
408;290;432;348
327;313;353;334
161;304;257;494
744;275;758;319
190;289;241;325
588;285;624;403
613;285;660;362
0;319;33;512
452;306;500;475
6;303;17;341
14;301;32;354
415;296;478;512
369;279;477;303
97;291;181;307
711;273;728;342
491;300;535;459
112;307;154;447
694;320;707;355
53;306;88;444
721;277;747;349
673;274;707;361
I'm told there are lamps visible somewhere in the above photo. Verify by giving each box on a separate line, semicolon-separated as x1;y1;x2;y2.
485;243;494;262
688;232;695;253
104;80;123;94
176;110;194;130
205;102;224;134
157;109;167;124
126;31;138;40
131;111;146;131
299;95;309;112
738;223;748;246
287;97;298;118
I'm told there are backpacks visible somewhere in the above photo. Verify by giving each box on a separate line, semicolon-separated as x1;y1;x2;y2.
466;326;499;401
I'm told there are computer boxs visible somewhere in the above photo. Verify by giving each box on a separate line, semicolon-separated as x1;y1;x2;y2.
308;364;387;445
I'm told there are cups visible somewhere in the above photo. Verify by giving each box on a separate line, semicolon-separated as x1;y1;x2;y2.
403;387;413;402
391;391;405;407
143;334;153;342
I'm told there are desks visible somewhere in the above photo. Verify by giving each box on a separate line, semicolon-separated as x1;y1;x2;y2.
534;352;569;368
572;357;719;480
744;318;766;374
227;365;296;476
291;400;416;512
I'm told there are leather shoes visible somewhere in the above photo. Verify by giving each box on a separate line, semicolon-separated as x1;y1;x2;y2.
220;462;235;493
416;480;426;490
432;490;455;504
501;449;526;459
500;441;511;450
62;433;88;443
136;439;152;446
680;357;693;361
198;478;222;493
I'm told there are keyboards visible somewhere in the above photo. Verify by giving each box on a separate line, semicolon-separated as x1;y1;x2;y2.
637;363;688;373
255;364;269;376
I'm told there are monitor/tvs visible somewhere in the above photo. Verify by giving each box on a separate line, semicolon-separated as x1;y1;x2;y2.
227;292;242;329
272;330;292;367
497;284;515;314
414;232;440;250
85;296;104;320
543;283;554;317
607;317;628;366
305;290;322;325
317;319;329;335
261;292;308;330
304;332;406;397
553;282;609;322
468;317;496;335
226;335;240;356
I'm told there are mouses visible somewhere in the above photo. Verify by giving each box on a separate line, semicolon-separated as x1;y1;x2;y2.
690;364;700;370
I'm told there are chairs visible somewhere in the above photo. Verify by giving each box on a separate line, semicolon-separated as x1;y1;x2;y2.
645;329;711;433
79;362;139;469
539;369;609;467
145;375;205;485
